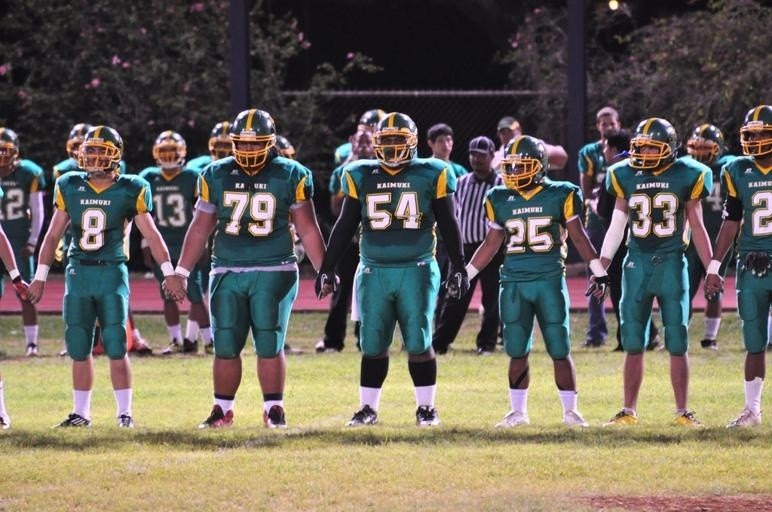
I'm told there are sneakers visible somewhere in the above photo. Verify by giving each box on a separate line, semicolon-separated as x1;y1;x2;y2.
494;409;530;429
345;403;379;427
115;410;134;428
0;416;11;429
198;404;235;428
124;337;215;357
674;411;706;428
602;409;639;428
562;409;590;427
25;340;38;358
262;405;288;429
415;405;441;428
725;406;762;429
49;413;91;429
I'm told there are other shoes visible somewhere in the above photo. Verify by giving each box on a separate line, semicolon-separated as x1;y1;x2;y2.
316;341;337;355
701;339;719;351
646;335;661;350
477;346;490;355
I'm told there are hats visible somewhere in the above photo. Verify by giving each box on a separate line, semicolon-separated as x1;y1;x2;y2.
467;136;496;155
496;116;520;134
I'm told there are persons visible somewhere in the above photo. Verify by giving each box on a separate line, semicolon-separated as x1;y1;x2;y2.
162;108;342;429
447;136;611;428
1;224;30;429
1;126;47;356
703;106;772;428
313;112;471;428
28;125;189;429
276;133;305;264
585;117;725;427
0;110;745;356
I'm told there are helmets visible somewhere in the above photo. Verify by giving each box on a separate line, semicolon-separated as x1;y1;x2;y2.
628;116;678;171
77;124;124;169
0;126;21;161
275;134;295;156
207;120;233;160
685;123;725;164
370;111;419;169
228;108;277;177
65;122;94;160
499;134;549;190
151;130;188;171
357;107;387;132
738;104;772;155
